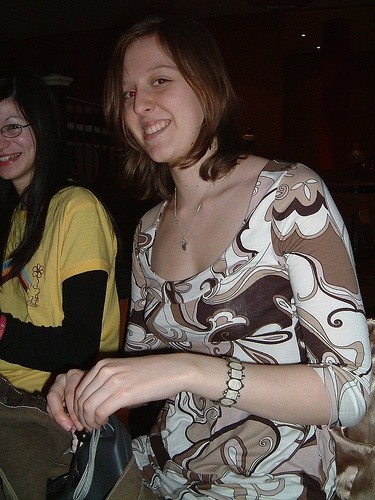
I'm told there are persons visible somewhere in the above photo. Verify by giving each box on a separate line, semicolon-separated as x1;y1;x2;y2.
46;18;373;500
0;72;120;500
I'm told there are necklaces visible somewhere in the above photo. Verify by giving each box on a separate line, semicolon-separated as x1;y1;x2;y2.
174;181;215;251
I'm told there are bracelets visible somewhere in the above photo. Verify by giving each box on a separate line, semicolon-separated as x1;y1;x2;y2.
210;357;247;409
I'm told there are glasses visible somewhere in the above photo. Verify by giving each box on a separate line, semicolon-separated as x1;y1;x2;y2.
0;119;30;140
